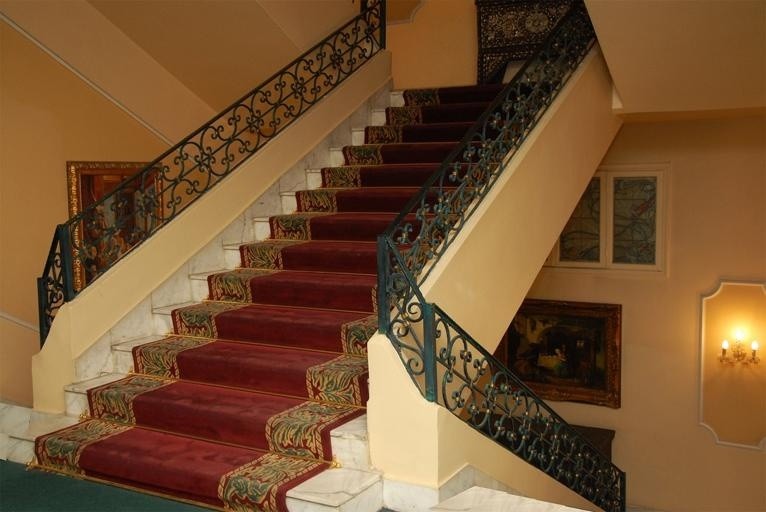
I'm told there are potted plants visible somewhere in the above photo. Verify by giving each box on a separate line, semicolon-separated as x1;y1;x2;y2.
490;298;622;409
66;160;163;317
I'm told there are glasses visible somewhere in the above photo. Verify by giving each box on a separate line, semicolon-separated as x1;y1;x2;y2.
718;327;762;367
